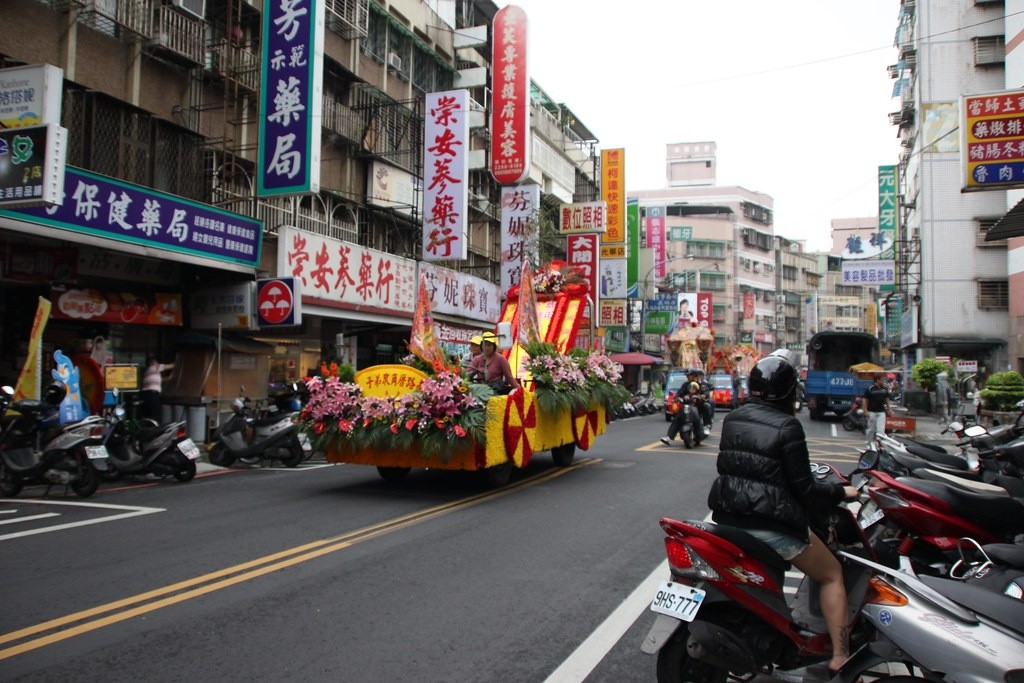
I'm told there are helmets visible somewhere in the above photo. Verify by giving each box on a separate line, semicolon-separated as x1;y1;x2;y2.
44;381;68;405
748;355;798;411
688;382;700;390
684;368;704;376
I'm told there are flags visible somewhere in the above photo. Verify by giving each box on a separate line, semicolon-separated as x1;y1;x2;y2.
410;277;446;370
519;257;541;349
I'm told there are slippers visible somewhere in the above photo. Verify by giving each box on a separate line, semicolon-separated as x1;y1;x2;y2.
826;665;864;683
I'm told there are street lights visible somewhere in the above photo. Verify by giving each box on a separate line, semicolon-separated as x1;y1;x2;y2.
638;254;696;389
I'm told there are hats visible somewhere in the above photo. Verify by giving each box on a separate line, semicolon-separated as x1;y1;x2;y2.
482;332;499;346
470;335;483;345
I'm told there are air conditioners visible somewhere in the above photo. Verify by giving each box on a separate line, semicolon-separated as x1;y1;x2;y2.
171;0;206;22
770;323;776;330
473;194;489;214
902;228;919;255
892;70;911;144
742;229;748;236
388;53;401;72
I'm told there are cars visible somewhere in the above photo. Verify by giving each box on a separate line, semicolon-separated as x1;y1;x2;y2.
662;367;709;418
711;376;750;409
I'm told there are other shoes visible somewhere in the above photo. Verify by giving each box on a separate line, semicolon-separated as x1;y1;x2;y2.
661;437;673;447
702;425;711;436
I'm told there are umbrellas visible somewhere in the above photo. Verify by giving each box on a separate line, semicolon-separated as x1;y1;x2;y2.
610;351;664;364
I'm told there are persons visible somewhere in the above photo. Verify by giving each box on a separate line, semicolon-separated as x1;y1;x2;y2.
466;330;516;393
141;357;176;424
662;367;715;446
460;335;483;368
708;348;865;683
862;374;893;450
678;300;693;318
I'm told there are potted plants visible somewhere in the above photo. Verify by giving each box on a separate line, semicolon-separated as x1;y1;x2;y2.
910;358;954;413
979;371;1024;434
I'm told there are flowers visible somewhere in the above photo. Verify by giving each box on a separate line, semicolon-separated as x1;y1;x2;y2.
521;340;632;414
532;261;581;293
294;348;496;464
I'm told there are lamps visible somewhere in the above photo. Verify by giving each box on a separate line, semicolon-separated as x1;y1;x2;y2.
913;295;922;303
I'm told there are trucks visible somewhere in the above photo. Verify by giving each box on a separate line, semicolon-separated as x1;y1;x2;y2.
803;330;882;420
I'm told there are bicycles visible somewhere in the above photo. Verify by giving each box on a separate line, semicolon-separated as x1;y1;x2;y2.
241;396;315;465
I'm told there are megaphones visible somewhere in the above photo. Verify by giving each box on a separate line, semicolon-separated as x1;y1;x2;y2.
913;295;921;303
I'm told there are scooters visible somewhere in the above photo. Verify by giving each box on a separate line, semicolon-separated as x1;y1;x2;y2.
637;395;1024;682
611;393;662;419
669;391;711;449
0;385;109;497
208;382;314;468
95;389;199;482
